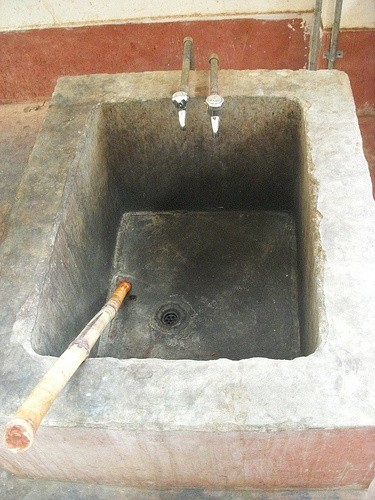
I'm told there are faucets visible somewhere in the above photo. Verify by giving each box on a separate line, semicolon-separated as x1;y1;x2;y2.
171;91;191;131
206;93;226;138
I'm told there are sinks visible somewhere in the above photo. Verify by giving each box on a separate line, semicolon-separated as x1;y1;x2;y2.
1;68;375;489
